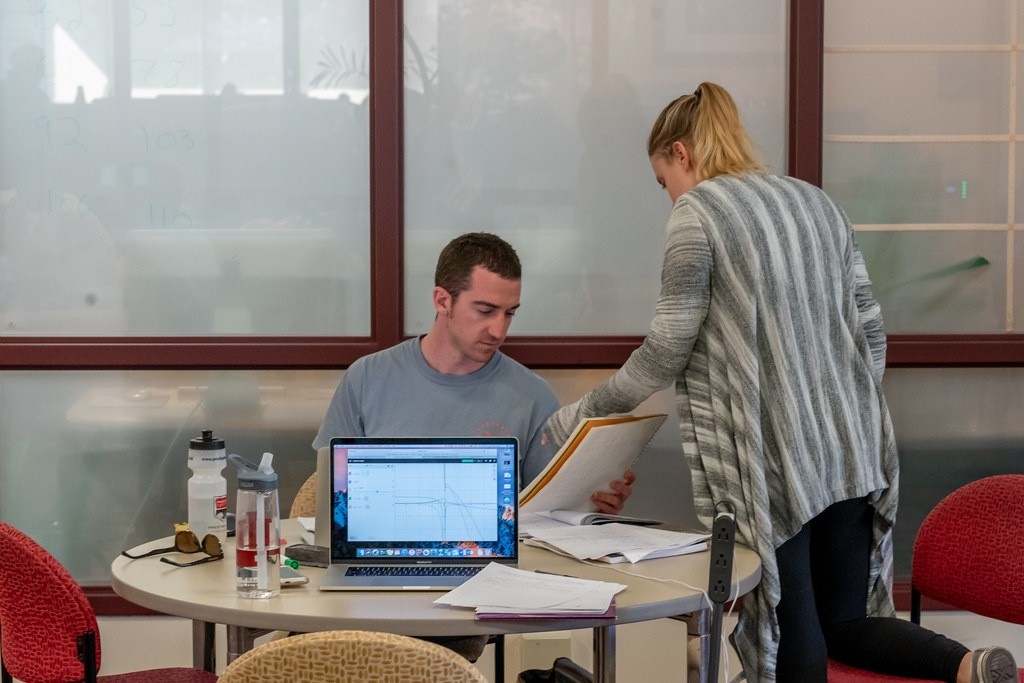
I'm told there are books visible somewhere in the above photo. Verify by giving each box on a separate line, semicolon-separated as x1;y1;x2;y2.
519;411;668;512
517;510;713;564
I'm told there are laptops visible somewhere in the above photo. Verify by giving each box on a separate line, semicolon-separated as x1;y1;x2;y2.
298;447;330;549
318;435;520;591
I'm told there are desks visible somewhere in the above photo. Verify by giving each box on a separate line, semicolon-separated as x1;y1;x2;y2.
111;516;762;683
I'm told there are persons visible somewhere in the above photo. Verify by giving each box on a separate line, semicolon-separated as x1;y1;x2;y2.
539;81;1019;683
316;230;565;665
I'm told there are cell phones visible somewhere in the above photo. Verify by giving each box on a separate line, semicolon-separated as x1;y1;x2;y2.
279;565;309;586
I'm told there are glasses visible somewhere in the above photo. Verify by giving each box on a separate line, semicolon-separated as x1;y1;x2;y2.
121;529;225;567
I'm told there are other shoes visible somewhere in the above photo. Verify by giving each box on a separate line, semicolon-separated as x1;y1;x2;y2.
971;646;1018;683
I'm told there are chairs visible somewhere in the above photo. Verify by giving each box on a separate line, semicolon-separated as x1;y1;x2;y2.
827;475;1024;683
0;522;219;683
217;630;490;683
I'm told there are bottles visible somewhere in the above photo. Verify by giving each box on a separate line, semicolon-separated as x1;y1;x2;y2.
229;452;282;598
187;429;227;546
